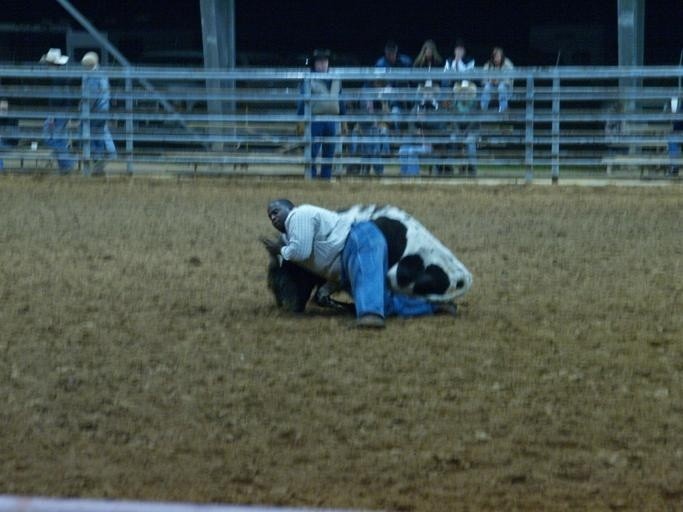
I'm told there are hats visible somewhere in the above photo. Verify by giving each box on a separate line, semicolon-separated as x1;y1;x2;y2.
420;79;440;90
82;51;99;66
306;47;333;65
453;82;478;96
40;49;69;65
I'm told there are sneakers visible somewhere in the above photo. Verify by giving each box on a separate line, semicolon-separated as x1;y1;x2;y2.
439;301;458;317
356;314;386;328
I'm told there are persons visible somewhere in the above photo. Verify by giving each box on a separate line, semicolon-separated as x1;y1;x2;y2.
662;95;683;175
76;50;111;176
257;196;459;329
0;101;19;170
38;47;75;175
295;35;517;180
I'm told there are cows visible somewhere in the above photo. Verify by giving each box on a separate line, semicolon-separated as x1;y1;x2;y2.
260;203;474;314
